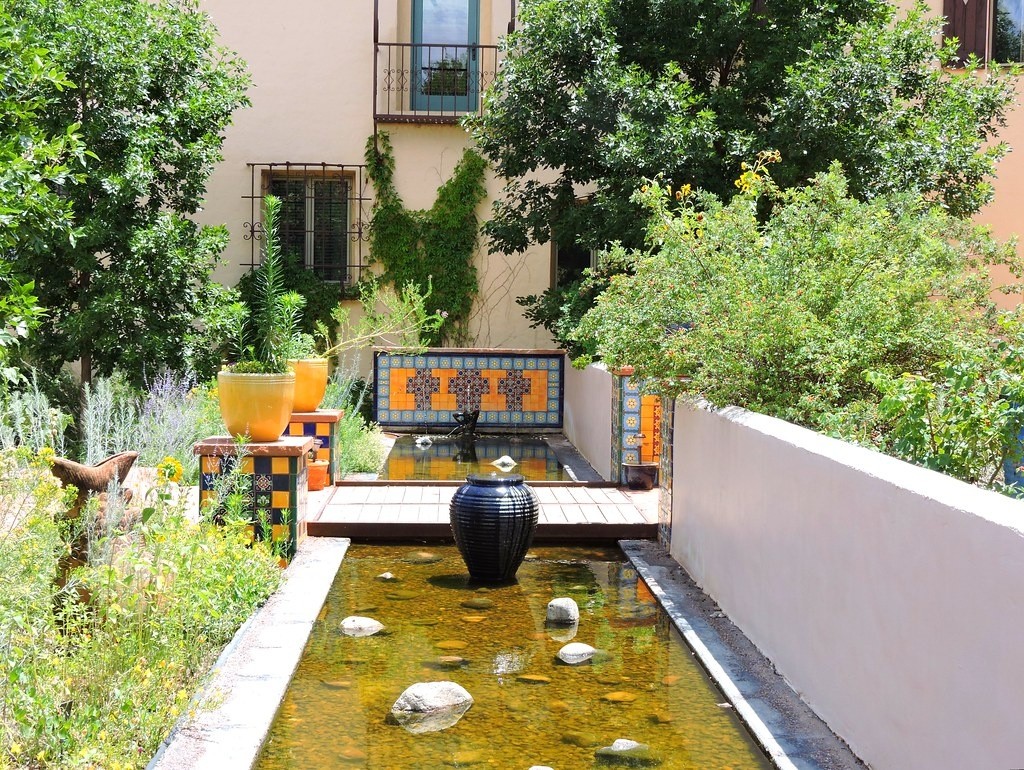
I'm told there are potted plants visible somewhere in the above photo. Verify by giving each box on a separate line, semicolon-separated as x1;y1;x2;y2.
209;195;305;443
279;275;450;414
307;439;329;491
